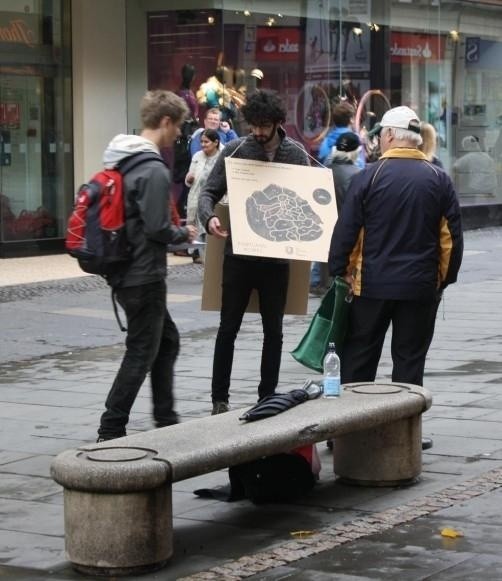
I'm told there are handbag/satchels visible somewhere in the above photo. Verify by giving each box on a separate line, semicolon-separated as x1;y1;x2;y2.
288;274;355;375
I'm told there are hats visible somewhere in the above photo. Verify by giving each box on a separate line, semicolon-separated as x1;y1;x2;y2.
365;105;421;138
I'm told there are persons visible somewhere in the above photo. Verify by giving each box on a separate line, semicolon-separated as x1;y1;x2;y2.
64;88;197;443
197;88;312;415
172;62;239;226
173;107;239;264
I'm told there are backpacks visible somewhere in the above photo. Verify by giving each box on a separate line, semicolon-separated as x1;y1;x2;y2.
65;151;169;280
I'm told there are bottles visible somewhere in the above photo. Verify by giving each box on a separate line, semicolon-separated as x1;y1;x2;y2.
323;342;342;400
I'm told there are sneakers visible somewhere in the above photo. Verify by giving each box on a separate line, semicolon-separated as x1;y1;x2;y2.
211;401;231;416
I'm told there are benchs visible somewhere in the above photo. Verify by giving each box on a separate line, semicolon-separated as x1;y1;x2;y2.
48;375;436;573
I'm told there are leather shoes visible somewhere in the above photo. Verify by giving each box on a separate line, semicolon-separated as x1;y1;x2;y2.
422;437;434;450
